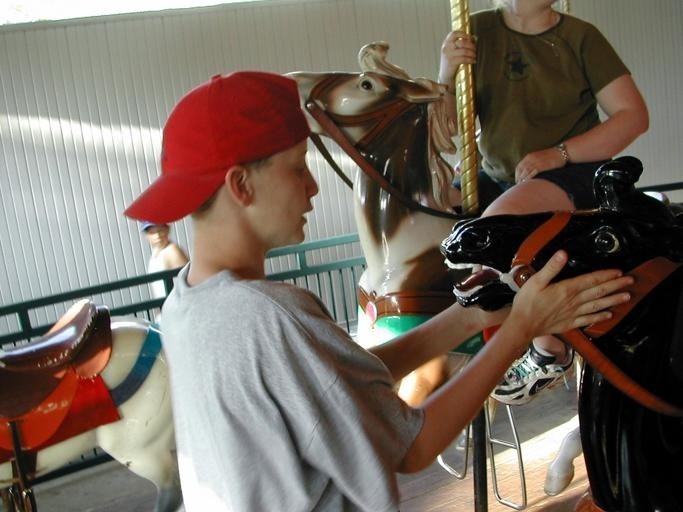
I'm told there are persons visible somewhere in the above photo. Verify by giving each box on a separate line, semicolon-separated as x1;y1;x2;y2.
435;0;651;409
139;219;190;298
122;69;638;510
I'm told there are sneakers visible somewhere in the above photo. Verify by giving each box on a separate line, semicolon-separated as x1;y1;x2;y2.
489;340;575;406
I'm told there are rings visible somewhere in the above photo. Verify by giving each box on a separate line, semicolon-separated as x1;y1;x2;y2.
453;39;463;51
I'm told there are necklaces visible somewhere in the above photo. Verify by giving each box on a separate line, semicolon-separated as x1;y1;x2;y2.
506;7;557;55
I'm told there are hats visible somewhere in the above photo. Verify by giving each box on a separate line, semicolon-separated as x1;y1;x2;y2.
124;71;311;225
139;221;162;231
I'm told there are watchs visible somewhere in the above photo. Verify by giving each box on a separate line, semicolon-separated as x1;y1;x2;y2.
550;143;570;168
